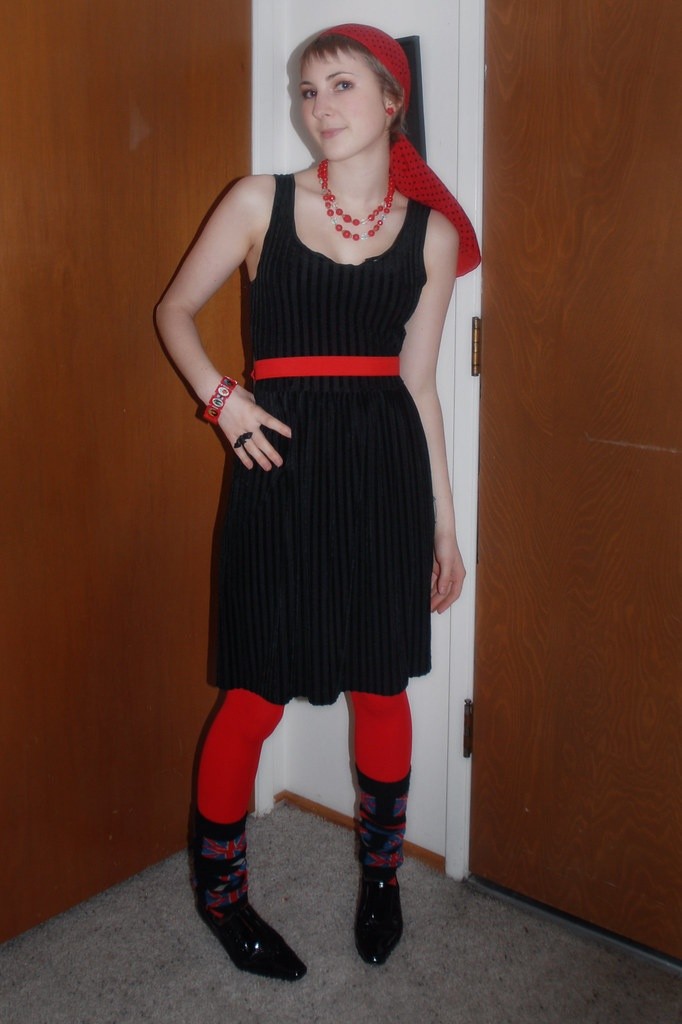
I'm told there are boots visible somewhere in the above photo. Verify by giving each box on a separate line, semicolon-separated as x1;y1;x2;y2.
354;764;411;965
195;809;307;982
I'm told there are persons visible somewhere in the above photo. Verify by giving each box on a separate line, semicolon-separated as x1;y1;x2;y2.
154;24;483;980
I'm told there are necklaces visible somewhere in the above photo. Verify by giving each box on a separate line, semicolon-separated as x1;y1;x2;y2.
318;158;396;242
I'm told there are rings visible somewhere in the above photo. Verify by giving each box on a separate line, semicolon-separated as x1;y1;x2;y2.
233;432;253;448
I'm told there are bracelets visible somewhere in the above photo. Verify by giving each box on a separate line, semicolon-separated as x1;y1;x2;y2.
202;377;238;426
